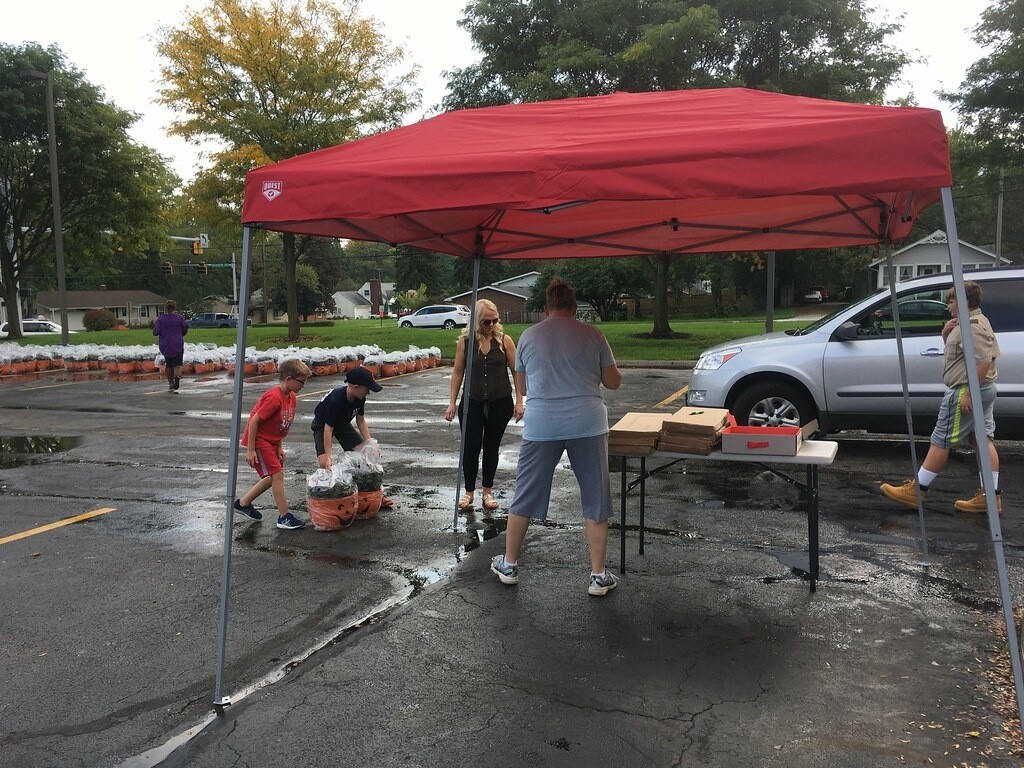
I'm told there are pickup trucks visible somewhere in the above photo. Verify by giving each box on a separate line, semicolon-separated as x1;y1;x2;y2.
369;311;397;320
184;311;253;329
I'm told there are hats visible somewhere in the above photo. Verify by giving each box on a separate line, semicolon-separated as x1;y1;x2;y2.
344;367;384;392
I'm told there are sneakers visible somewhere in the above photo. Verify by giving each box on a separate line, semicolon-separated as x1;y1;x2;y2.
587;569;619;595
880;478;926;510
276;512;305;529
491;555;519;585
953;487;1003;515
233;499;263;520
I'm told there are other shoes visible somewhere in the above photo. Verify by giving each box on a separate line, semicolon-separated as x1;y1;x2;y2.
381;494;394;506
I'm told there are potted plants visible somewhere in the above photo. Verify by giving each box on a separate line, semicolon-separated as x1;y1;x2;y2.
307;472;384;530
0;353;441;377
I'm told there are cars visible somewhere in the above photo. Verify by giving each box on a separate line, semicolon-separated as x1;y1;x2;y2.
399;309;416;317
0;313;80;339
803;287;830;305
683;263;1024;446
396;304;471;330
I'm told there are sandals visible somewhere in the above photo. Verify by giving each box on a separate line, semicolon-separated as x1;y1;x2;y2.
459;495;474;509
483;494;499;509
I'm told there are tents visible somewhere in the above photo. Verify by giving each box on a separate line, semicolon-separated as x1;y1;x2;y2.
214;88;1024;735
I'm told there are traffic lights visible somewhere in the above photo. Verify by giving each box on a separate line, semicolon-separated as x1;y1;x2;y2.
163;261;172;275
191;241;201;256
198;261;207;275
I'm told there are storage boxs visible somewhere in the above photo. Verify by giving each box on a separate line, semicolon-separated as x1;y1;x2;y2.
609;406;819;456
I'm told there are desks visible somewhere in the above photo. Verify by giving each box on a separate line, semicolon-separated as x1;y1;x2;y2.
608;440;838;593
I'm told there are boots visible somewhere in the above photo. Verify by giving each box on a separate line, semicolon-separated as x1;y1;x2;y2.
166;367;176;390
174;365;183;390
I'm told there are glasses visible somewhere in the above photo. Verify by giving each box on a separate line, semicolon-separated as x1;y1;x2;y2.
285;375;306;386
481;317;498;325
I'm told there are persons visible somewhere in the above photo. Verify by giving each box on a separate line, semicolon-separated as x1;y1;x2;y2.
153;300;189;390
311;368;394;506
235;358;312;529
38;315;51;331
491;277;621;595
444;299;524;509
879;282;1003;512
